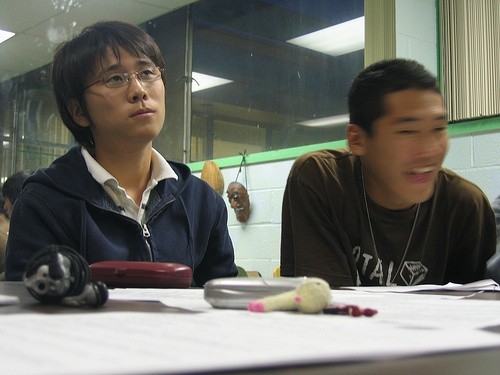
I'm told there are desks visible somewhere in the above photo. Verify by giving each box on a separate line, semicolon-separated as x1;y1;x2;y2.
0;280;500;375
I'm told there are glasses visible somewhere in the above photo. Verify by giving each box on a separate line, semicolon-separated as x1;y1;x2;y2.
80;64;165;91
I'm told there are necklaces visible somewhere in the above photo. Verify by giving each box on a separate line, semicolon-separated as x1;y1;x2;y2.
360;156;420;287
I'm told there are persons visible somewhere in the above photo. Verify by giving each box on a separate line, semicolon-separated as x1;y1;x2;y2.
280;58;497;290
1;169;32;218
4;21;239;287
